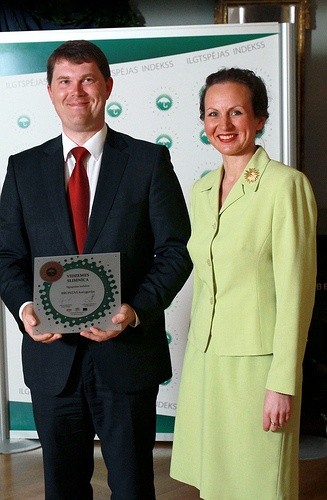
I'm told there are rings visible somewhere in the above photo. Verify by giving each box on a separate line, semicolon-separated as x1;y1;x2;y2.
270;421;278;427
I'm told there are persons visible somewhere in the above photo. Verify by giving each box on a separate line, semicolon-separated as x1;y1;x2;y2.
0;39;194;499
168;67;317;499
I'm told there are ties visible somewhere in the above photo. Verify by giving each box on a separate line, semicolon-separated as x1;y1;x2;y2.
65;148;91;254
